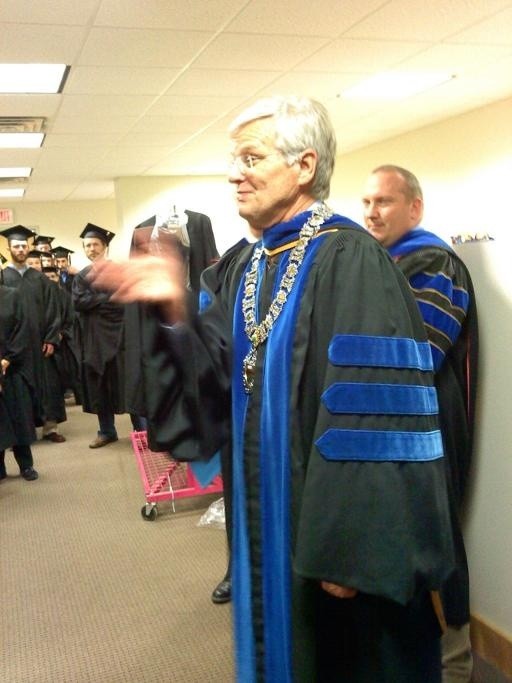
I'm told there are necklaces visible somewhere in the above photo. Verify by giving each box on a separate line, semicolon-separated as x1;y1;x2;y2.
241;202;334;393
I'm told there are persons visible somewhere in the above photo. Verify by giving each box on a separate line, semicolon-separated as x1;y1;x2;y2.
361;164;479;682
0;224;68;443
122;208;222;416
87;96;456;683
0;253;40;480
25;249;43;275
71;223;148;452
49;247;75;295
40;252;55;267
32;235;53;253
42;266;74;396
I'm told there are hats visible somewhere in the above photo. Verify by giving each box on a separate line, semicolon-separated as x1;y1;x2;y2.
26;251;41;257
0;225;36;240
80;223;115;246
32;236;54;245
41;252;50;258
0;253;7;264
43;267;56;273
50;246;73;258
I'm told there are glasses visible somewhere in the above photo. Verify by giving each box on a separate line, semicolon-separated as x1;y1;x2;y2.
231;148;289;174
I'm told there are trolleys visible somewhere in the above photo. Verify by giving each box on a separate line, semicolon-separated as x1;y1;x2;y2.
131;431;226;522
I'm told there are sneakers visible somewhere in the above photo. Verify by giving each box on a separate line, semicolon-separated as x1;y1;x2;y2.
20;465;38;480
89;432;117;447
0;471;7;479
442;625;472;666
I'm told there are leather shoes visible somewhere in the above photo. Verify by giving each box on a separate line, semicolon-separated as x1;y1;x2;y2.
45;433;64;442
211;574;232;603
